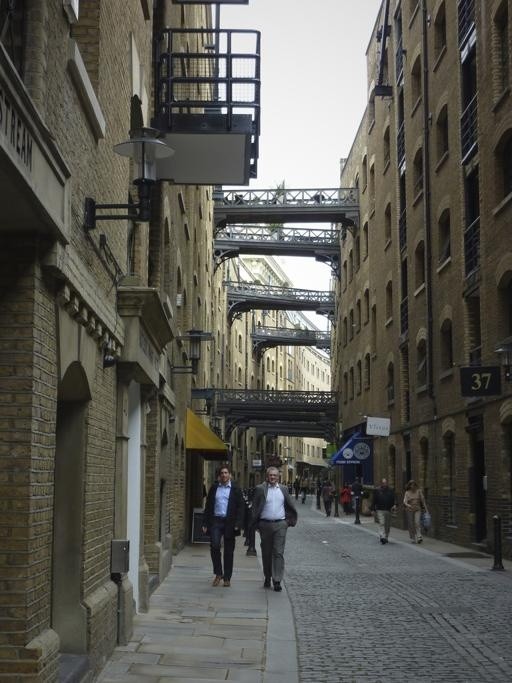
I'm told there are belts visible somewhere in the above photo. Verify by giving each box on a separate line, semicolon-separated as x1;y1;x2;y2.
260;519;287;523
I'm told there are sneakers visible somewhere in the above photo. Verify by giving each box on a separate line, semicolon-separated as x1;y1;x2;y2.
412;540;415;543
418;537;422;544
213;575;223;585
380;538;388;544
223;579;230;586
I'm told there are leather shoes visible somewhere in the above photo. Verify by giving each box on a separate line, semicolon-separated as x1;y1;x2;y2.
264;578;271;586
274;582;282;591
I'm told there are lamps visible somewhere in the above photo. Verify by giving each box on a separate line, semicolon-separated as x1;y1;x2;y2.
172;327;215;373
494;346;511;381
84;125;175;227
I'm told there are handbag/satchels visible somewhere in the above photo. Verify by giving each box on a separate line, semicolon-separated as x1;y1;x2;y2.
423;511;432;530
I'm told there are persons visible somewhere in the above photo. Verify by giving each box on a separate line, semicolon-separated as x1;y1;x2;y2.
352;476;363;495
294;476;299;499
371;478;397;544
403;479;428;543
248;466;298;590
339;481;353;514
299;476;308;503
320;479;334;517
202;465;249;587
